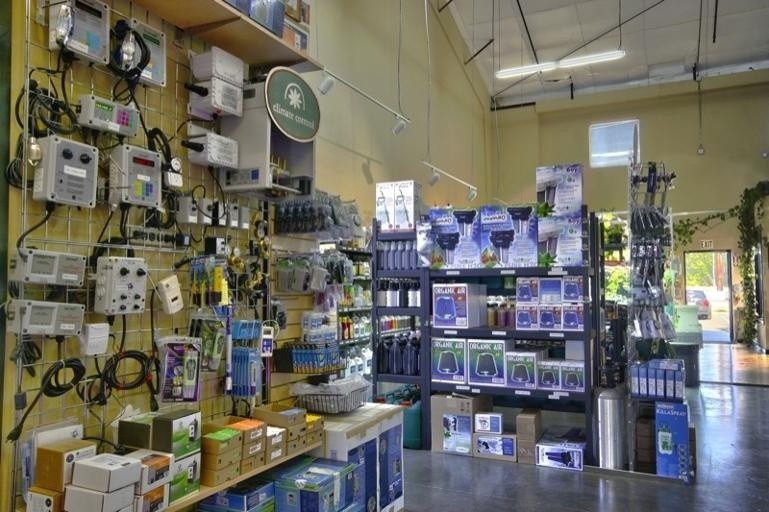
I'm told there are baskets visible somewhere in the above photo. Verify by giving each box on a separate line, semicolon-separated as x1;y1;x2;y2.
293;385;370;415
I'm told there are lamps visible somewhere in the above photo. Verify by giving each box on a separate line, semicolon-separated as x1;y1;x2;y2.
312;0;413;138
417;0;479;202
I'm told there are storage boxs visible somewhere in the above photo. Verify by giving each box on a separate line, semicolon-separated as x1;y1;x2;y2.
222;0;311;56
636;400;698;482
417;159;592;474
375;180;425;234
9;395;405;512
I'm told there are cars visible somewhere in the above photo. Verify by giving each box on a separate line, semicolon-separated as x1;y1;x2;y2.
686;289;713;319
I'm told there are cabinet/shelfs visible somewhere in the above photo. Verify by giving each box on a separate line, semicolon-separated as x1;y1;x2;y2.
280;344;372;415
334;244;372;384
370;217;430;448
